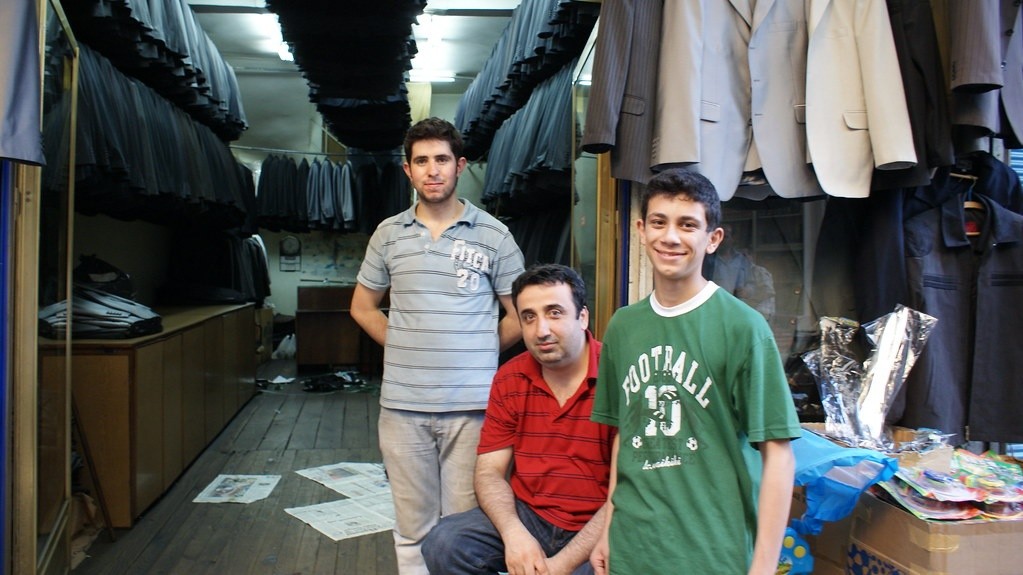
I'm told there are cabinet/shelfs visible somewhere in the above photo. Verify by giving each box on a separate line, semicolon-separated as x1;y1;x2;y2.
37;301;258;530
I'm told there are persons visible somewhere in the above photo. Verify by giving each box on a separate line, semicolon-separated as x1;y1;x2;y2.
351;118;528;575
590;169;803;575
420;263;608;575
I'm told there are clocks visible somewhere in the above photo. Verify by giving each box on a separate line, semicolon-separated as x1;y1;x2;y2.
279;235;300;257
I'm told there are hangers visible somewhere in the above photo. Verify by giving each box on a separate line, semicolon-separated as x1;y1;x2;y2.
959;174;985;236
265;151;403;169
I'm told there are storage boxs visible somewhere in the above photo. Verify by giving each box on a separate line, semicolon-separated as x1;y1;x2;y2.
256;306;274;365
785;422;1023;575
